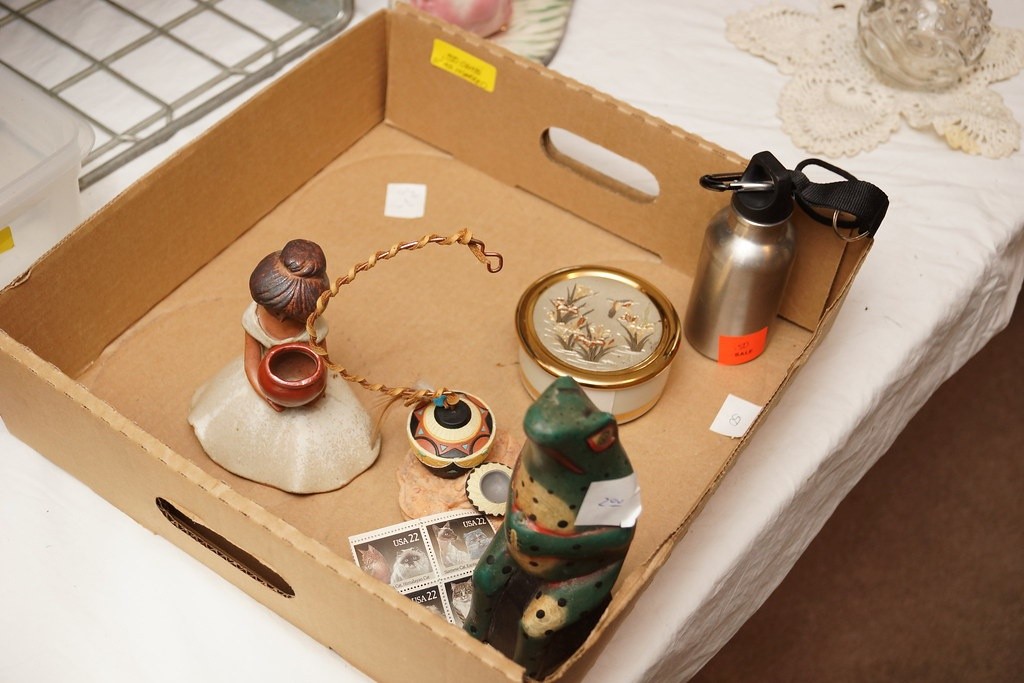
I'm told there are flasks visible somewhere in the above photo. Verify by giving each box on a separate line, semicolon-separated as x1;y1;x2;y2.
683;151;885;365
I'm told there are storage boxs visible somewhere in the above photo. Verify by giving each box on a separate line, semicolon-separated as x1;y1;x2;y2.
0;2;890;681
0;59;96;294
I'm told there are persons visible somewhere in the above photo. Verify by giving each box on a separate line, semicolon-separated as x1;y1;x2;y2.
185;236;381;495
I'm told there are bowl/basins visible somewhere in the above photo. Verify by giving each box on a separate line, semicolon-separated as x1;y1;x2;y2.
405;389;497;480
